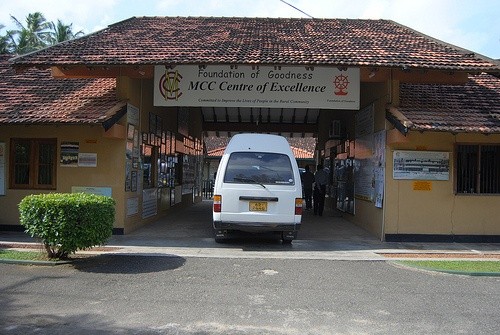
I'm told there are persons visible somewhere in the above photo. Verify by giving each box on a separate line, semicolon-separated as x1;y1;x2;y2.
300;165;315;209
313;165;330;217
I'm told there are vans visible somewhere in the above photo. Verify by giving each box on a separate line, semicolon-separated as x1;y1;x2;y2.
212;133;306;246
